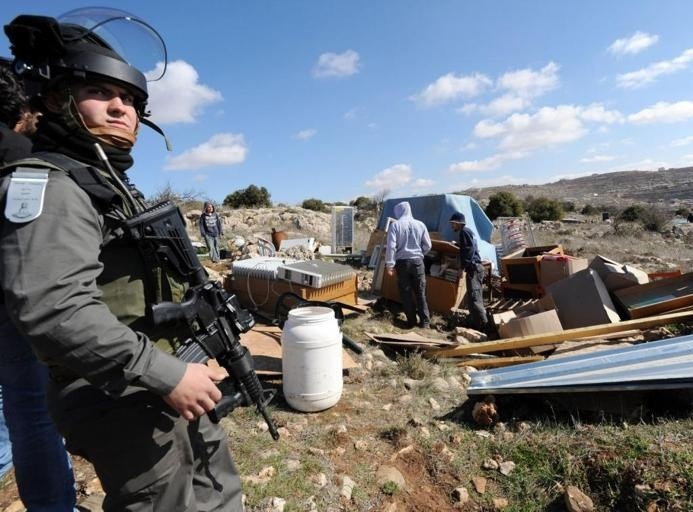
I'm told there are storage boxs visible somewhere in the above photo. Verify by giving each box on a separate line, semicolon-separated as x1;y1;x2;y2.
381;240;468;311
539;255;588;286
544;268;621;330
492;309;567;354
589;254;649;289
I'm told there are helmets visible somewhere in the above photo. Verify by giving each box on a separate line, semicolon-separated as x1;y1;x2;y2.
3;5;173;176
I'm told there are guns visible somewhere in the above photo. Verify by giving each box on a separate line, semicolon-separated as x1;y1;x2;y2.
119;199;280;442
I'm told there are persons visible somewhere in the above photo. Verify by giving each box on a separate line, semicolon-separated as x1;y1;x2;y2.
197;201;224;264
448;212;488;330
384;201;432;329
294;218;301;228
0;56;75;511
1;7;242;511
270;228;278;251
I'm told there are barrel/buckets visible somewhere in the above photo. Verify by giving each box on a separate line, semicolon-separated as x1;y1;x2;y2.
283;307;344;413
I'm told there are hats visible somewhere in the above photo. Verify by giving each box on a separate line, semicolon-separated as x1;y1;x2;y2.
448;211;467;225
